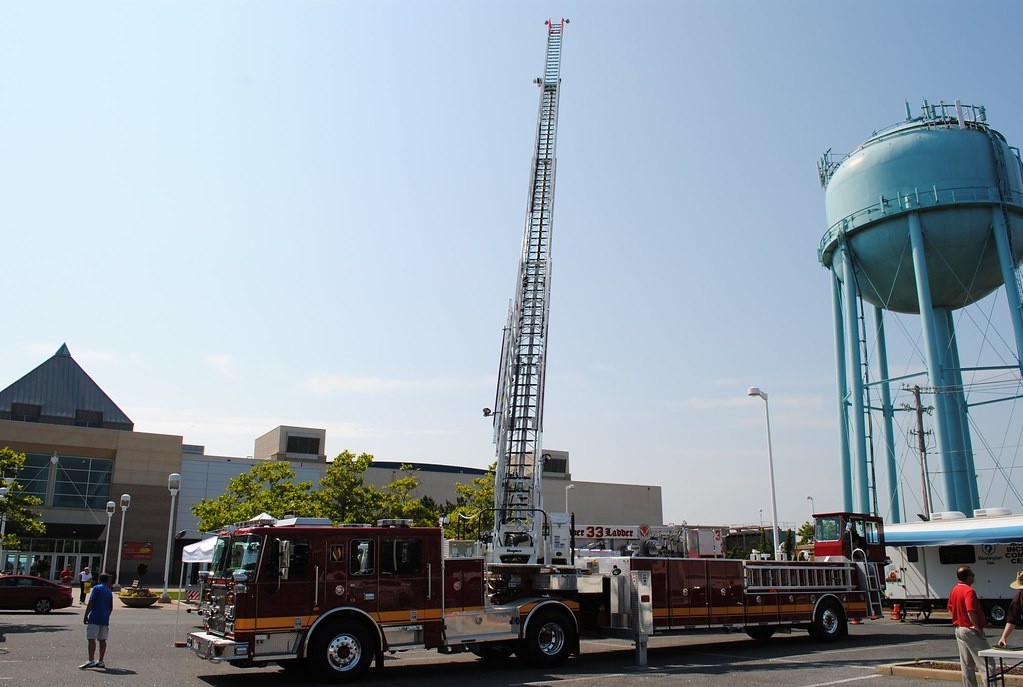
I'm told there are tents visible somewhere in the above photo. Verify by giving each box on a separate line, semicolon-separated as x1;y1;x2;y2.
172;537;224;649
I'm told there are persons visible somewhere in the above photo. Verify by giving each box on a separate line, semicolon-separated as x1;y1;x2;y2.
79;573;113;668
799;552;805;561
79;568;92;605
947;565;997;687
997;571;1023;647
60;563;73;586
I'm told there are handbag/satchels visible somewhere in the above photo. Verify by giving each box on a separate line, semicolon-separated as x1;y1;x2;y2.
83;582;91;593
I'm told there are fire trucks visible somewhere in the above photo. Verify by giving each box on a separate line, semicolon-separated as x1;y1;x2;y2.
569;509;892;672
174;16;628;687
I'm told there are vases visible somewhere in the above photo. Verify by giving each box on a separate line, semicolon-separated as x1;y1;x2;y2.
119;596;160;607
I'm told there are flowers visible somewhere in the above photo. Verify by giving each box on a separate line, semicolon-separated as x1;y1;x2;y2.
118;588;156;598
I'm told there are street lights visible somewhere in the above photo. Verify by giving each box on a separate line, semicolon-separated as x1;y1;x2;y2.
161;472;181;597
806;495;814;514
746;387;780;561
101;501;116;574
115;493;132;585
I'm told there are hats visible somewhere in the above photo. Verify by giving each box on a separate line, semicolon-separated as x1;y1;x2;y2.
1009;572;1023;589
84;567;89;570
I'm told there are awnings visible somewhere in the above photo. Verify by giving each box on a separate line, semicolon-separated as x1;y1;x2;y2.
4;520;106;540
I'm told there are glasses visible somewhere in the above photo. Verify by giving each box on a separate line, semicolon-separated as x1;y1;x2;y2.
968;573;974;577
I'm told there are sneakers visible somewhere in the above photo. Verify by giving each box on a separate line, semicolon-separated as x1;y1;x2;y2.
78;660;96;668
94;660;105;668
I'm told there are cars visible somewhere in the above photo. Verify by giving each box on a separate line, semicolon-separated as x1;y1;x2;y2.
0;574;74;615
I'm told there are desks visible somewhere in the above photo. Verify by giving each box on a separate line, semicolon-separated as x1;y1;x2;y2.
978;648;1023;687
